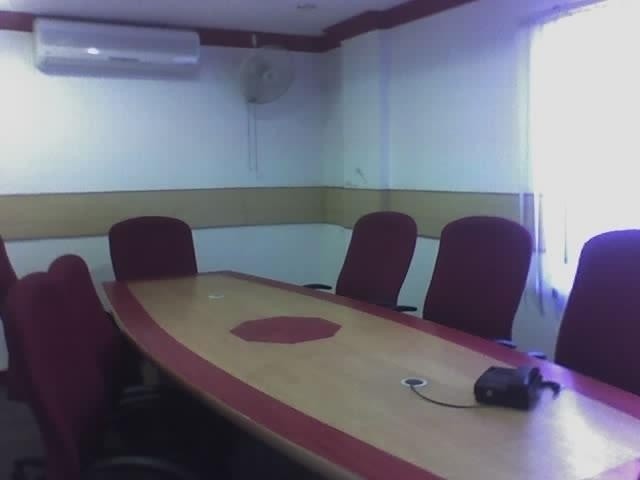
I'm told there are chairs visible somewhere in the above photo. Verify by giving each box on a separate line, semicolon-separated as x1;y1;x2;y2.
395;217;533;347
301;211;422;318
0;241;224;478
109;216;199;281
524;229;640;400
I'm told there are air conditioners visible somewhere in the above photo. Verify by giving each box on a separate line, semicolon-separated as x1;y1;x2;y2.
32;17;201;73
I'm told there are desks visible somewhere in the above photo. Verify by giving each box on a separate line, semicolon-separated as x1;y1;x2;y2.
101;271;639;478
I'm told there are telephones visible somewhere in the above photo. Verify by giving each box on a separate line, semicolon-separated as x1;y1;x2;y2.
474;366;542;411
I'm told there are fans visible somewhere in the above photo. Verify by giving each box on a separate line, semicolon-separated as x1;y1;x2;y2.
238;41;293;103
